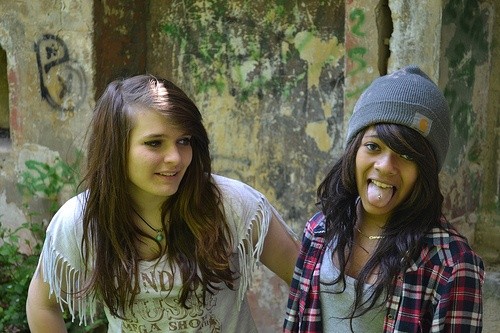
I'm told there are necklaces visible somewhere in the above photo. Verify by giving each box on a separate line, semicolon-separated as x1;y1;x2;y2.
126;191;170;241
350;197;406;241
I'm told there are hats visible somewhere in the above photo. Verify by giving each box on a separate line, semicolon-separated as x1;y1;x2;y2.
344;65;451;170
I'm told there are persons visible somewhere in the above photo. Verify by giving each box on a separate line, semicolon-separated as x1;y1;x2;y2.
25;75;318;333
283;61;487;333
